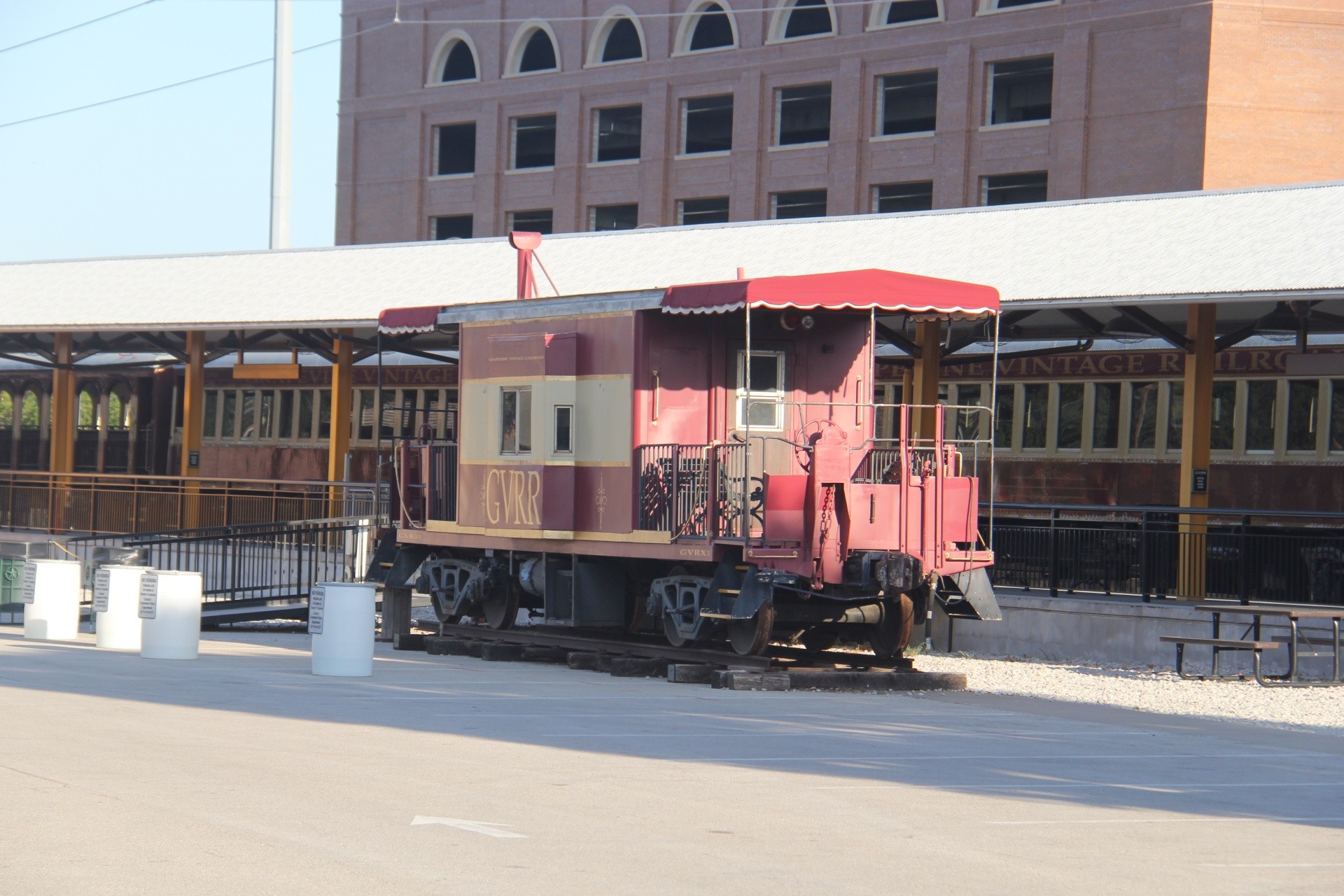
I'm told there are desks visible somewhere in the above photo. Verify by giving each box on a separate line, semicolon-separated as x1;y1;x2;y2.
1193;605;1344;686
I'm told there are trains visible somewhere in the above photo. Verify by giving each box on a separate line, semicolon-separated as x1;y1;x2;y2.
375;226;1004;687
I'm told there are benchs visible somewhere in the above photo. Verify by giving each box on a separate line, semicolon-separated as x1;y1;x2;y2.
1158;636;1281;688
1271;636;1344;687
